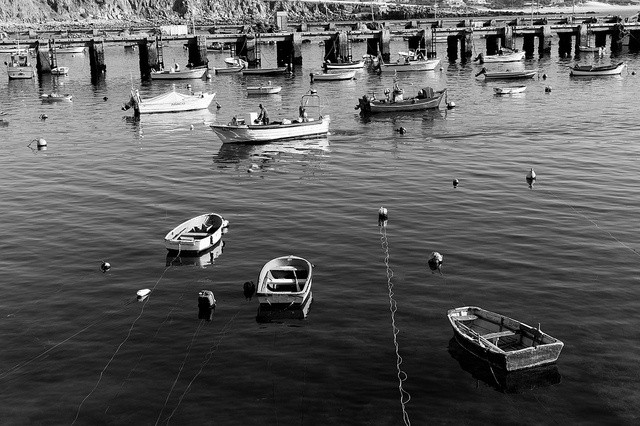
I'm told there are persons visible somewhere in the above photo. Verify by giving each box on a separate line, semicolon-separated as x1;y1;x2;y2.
227;117;238;126
253;103;270;126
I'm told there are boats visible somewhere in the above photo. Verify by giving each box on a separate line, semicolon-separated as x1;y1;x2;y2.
310;71;356;80
483;50;526;63
484;70;536;79
247;85;281;94
256;255;312;305
493;85;526;94
164;213;225;253
135;90;216;113
4;52;36;79
371;59;440;71
51;65;69;75
571;61;625;76
208;64;243;73
210;91;330;143
323;58;365;68
448;306;564;374
150;67;207;79
41;93;73;102
358;68;446;112
224;58;249;69
243;67;287;74
